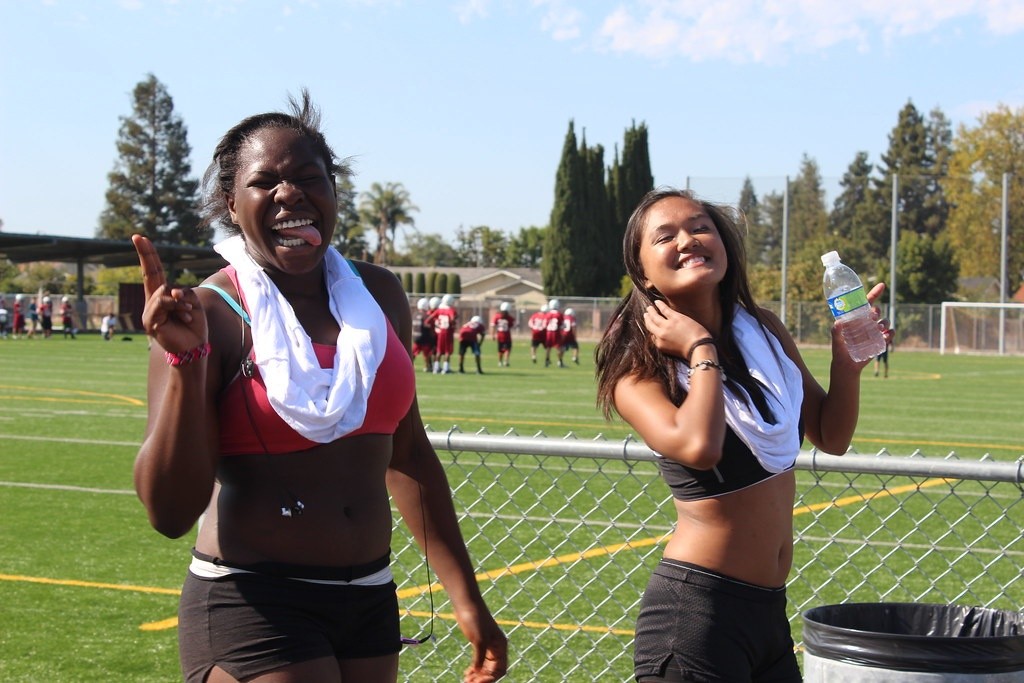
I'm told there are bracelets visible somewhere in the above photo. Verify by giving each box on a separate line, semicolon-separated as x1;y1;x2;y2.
686;358;727;389
687;337;715;364
165;341;211;365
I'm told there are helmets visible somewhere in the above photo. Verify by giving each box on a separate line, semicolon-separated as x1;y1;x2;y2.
442;294;455;304
62;297;68;302
16;294;23;300
44;297;51;302
471;316;482;324
541;304;549;312
548;299;561;310
429;297;441;308
500;302;510;311
564;308;575;316
417;298;429;309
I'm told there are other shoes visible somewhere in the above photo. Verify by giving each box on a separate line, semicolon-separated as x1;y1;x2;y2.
446;370;451;373
573;358;578;363
460;369;465;373
545;358;549;366
478;368;483;373
504;362;509;367
436;369;442;373
533;356;537;363
558;361;563;367
498;363;502;367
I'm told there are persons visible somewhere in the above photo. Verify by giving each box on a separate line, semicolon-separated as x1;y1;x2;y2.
26;295;38;338
593;184;894;683
100;313;115;341
413;293;460;374
561;308;579;363
59;296;77;340
36;290;53;338
0;293;9;339
77;297;88;333
490;302;516;368
545;299;565;367
458;316;486;374
528;304;551;364
874;329;894;377
12;294;26;339
132;86;509;683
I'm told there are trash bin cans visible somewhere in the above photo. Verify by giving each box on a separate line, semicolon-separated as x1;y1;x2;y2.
802;603;1024;683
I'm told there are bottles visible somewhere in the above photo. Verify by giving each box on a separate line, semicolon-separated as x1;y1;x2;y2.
821;250;886;361
